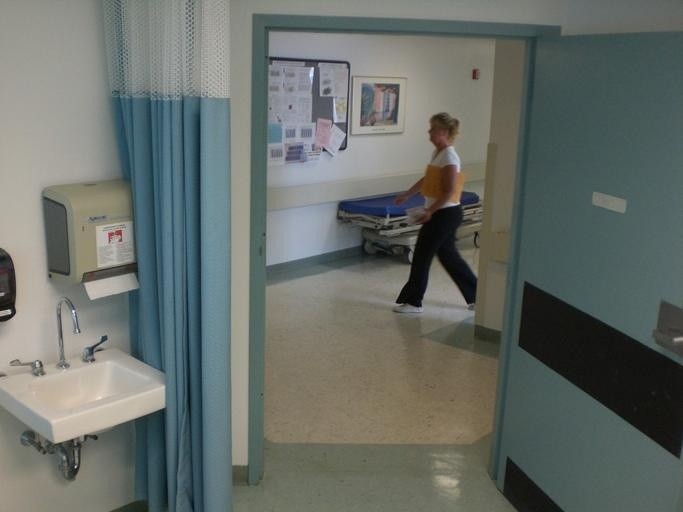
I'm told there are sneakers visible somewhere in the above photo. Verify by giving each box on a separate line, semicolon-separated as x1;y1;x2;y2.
392;302;424;313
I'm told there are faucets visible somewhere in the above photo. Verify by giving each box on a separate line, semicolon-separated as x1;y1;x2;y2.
56;297;81;368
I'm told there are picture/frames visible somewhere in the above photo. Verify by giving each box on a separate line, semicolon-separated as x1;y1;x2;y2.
349;74;408;135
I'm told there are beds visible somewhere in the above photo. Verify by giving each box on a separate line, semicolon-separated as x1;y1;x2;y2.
336;188;484;264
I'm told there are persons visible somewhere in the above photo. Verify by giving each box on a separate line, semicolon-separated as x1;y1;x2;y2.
390;110;482;317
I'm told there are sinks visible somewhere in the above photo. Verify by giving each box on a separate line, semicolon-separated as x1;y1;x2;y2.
0;348;166;445
